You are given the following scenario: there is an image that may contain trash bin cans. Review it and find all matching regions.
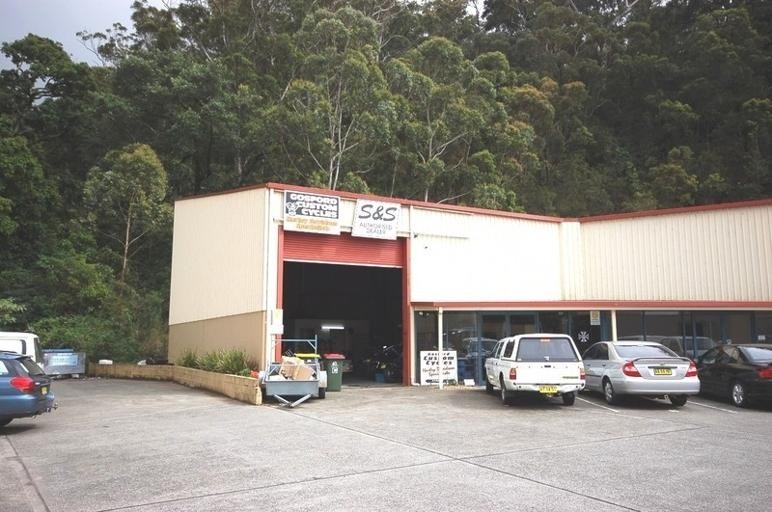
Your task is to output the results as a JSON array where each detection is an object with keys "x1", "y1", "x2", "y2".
[{"x1": 323, "y1": 354, "x2": 346, "y2": 392}]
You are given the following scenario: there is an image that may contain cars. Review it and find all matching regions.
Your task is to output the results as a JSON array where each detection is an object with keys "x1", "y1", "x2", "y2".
[
  {"x1": 0, "y1": 350, "x2": 58, "y2": 429},
  {"x1": 484, "y1": 333, "x2": 772, "y2": 408},
  {"x1": 461, "y1": 337, "x2": 497, "y2": 357}
]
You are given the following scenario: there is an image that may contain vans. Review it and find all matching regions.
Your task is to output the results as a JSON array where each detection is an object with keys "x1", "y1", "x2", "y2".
[{"x1": 0, "y1": 331, "x2": 45, "y2": 372}]
